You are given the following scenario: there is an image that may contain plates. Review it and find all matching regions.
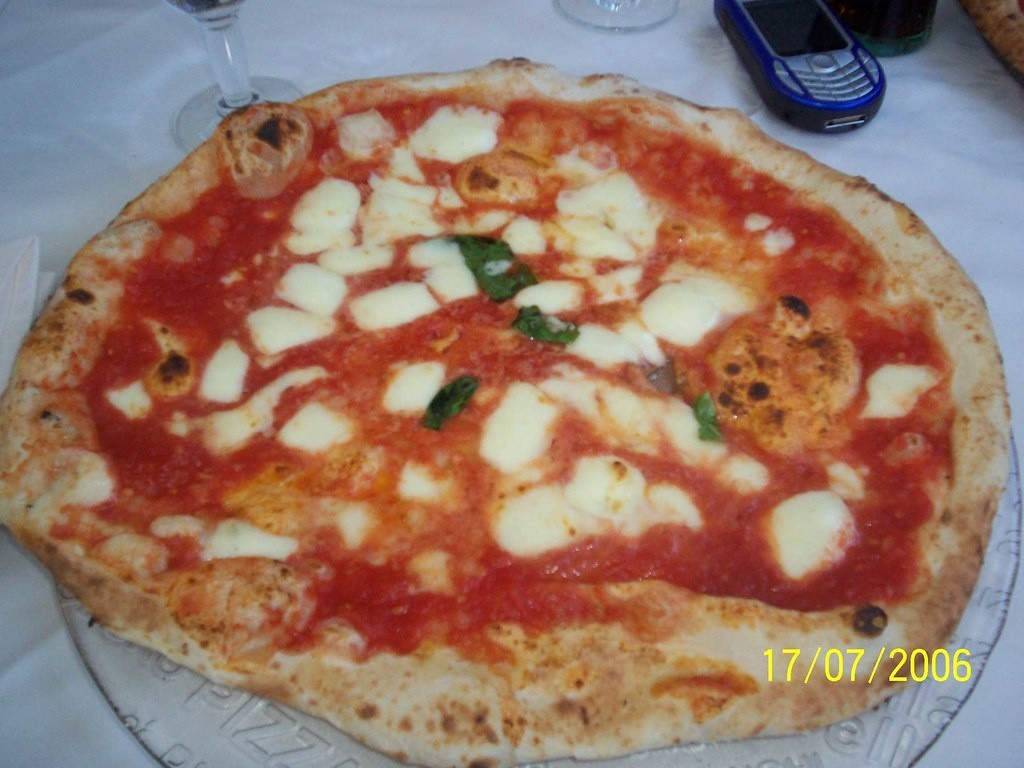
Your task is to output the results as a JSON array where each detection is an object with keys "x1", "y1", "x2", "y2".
[{"x1": 52, "y1": 425, "x2": 1022, "y2": 768}]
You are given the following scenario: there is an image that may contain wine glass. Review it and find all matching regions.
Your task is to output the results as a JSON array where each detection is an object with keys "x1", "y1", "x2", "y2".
[{"x1": 165, "y1": 0, "x2": 303, "y2": 157}]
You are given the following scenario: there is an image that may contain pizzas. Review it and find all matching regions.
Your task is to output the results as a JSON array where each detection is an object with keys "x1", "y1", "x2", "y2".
[
  {"x1": 961, "y1": 0, "x2": 1024, "y2": 76},
  {"x1": 1, "y1": 60, "x2": 1011, "y2": 765}
]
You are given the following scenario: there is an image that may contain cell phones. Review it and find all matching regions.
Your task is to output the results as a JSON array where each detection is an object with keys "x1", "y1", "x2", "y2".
[
  {"x1": 824, "y1": 0, "x2": 935, "y2": 56},
  {"x1": 714, "y1": 0, "x2": 887, "y2": 134}
]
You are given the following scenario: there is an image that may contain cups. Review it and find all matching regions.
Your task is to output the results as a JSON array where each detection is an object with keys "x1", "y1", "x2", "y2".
[{"x1": 828, "y1": 0, "x2": 939, "y2": 60}]
